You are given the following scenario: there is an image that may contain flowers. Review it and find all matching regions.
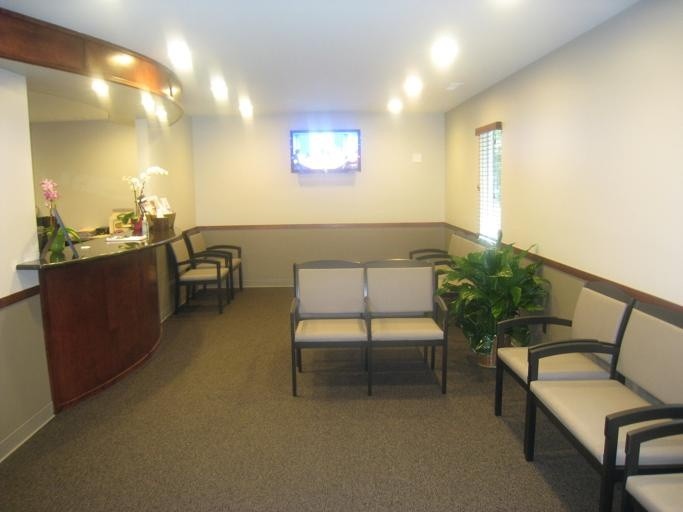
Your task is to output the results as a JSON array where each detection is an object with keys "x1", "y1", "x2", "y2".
[
  {"x1": 115, "y1": 165, "x2": 168, "y2": 225},
  {"x1": 35, "y1": 177, "x2": 82, "y2": 244}
]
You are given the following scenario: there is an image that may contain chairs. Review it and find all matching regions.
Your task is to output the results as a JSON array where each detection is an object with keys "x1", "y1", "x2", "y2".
[
  {"x1": 166, "y1": 227, "x2": 243, "y2": 316},
  {"x1": 494, "y1": 281, "x2": 634, "y2": 460},
  {"x1": 365, "y1": 259, "x2": 449, "y2": 398},
  {"x1": 408, "y1": 235, "x2": 488, "y2": 328},
  {"x1": 289, "y1": 259, "x2": 371, "y2": 396}
]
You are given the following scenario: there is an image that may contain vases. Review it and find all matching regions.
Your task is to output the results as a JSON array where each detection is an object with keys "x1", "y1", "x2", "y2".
[
  {"x1": 129, "y1": 216, "x2": 143, "y2": 236},
  {"x1": 44, "y1": 231, "x2": 64, "y2": 252}
]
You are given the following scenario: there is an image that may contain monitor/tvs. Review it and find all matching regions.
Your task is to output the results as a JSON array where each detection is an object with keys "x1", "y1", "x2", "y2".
[{"x1": 290, "y1": 128, "x2": 362, "y2": 174}]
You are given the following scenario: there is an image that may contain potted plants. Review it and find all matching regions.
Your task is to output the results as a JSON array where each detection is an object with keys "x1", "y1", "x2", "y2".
[{"x1": 433, "y1": 228, "x2": 554, "y2": 368}]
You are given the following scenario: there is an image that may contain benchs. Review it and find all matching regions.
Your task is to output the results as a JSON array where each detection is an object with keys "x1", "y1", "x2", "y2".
[{"x1": 525, "y1": 300, "x2": 683, "y2": 510}]
